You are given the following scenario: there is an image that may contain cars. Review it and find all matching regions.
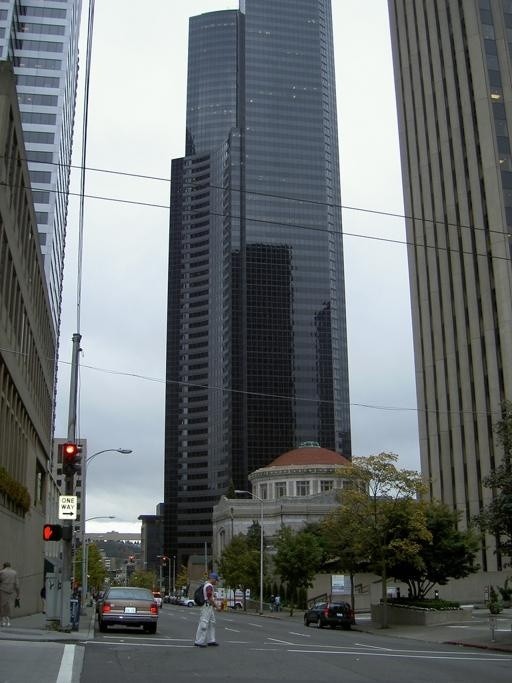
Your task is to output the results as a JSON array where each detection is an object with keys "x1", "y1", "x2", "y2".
[
  {"x1": 95, "y1": 590, "x2": 105, "y2": 600},
  {"x1": 99, "y1": 585, "x2": 158, "y2": 634},
  {"x1": 96, "y1": 597, "x2": 104, "y2": 609},
  {"x1": 153, "y1": 591, "x2": 199, "y2": 608}
]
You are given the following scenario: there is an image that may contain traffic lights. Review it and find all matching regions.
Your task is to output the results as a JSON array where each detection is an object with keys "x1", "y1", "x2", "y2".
[
  {"x1": 41, "y1": 522, "x2": 63, "y2": 541},
  {"x1": 60, "y1": 442, "x2": 79, "y2": 475}
]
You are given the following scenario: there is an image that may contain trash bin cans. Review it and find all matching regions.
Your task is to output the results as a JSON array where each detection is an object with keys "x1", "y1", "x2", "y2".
[{"x1": 70, "y1": 600, "x2": 80, "y2": 631}]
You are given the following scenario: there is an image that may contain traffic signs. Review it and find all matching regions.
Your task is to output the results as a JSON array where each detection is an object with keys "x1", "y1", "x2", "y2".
[{"x1": 57, "y1": 494, "x2": 78, "y2": 521}]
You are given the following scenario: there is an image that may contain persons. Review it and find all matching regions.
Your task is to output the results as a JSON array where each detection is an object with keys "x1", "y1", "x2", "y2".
[
  {"x1": 194, "y1": 573, "x2": 221, "y2": 648},
  {"x1": 269, "y1": 595, "x2": 275, "y2": 613},
  {"x1": 0, "y1": 561, "x2": 20, "y2": 627},
  {"x1": 274, "y1": 594, "x2": 280, "y2": 613}
]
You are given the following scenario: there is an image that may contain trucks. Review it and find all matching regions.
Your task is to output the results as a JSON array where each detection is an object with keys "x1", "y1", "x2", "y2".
[{"x1": 212, "y1": 587, "x2": 251, "y2": 609}]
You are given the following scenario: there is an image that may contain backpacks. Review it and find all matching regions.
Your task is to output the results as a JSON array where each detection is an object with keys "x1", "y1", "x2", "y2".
[{"x1": 194, "y1": 584, "x2": 206, "y2": 606}]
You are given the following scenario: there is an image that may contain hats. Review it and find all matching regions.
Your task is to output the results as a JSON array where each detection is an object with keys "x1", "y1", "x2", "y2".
[{"x1": 210, "y1": 573, "x2": 221, "y2": 581}]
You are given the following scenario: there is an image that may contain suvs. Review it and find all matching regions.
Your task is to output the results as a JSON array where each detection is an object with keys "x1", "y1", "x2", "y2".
[{"x1": 303, "y1": 599, "x2": 353, "y2": 629}]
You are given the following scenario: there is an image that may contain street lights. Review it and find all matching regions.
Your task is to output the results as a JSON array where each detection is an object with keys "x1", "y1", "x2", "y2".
[
  {"x1": 85, "y1": 534, "x2": 103, "y2": 594},
  {"x1": 80, "y1": 447, "x2": 133, "y2": 601},
  {"x1": 234, "y1": 489, "x2": 265, "y2": 615},
  {"x1": 156, "y1": 555, "x2": 171, "y2": 596},
  {"x1": 72, "y1": 515, "x2": 116, "y2": 600}
]
